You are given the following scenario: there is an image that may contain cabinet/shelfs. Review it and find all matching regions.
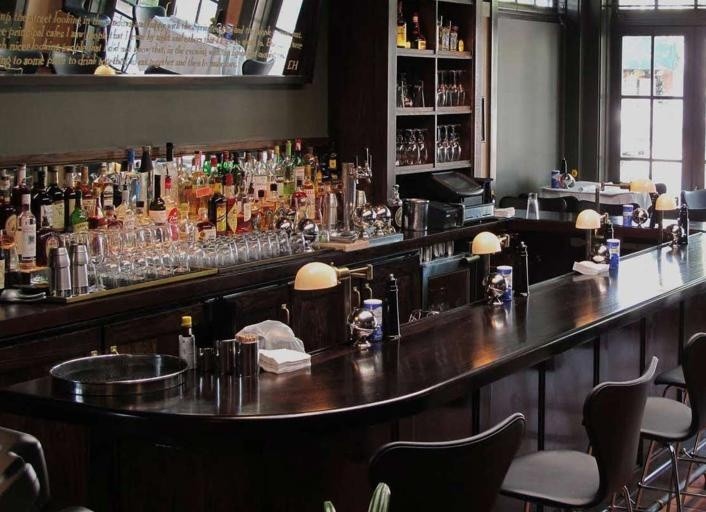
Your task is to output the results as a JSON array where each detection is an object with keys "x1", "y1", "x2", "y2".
[
  {"x1": 324, "y1": 0, "x2": 478, "y2": 230},
  {"x1": 0, "y1": 252, "x2": 422, "y2": 512}
]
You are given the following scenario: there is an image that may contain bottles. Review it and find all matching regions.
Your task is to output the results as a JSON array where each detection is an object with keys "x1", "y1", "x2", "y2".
[
  {"x1": 512, "y1": 241, "x2": 531, "y2": 297},
  {"x1": 387, "y1": 185, "x2": 403, "y2": 233},
  {"x1": 678, "y1": 203, "x2": 689, "y2": 245},
  {"x1": 396, "y1": 1, "x2": 428, "y2": 49},
  {"x1": 0, "y1": 137, "x2": 340, "y2": 294},
  {"x1": 438, "y1": 15, "x2": 465, "y2": 52},
  {"x1": 395, "y1": 71, "x2": 426, "y2": 107},
  {"x1": 177, "y1": 315, "x2": 196, "y2": 370},
  {"x1": 382, "y1": 273, "x2": 401, "y2": 340},
  {"x1": 526, "y1": 192, "x2": 542, "y2": 220},
  {"x1": 560, "y1": 157, "x2": 567, "y2": 174}
]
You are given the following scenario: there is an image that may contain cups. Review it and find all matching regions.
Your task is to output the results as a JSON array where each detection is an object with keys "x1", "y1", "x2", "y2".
[
  {"x1": 364, "y1": 298, "x2": 383, "y2": 342},
  {"x1": 496, "y1": 265, "x2": 514, "y2": 302},
  {"x1": 198, "y1": 339, "x2": 259, "y2": 379},
  {"x1": 48, "y1": 221, "x2": 306, "y2": 299},
  {"x1": 622, "y1": 205, "x2": 634, "y2": 228},
  {"x1": 606, "y1": 238, "x2": 621, "y2": 270},
  {"x1": 223, "y1": 24, "x2": 233, "y2": 47},
  {"x1": 550, "y1": 169, "x2": 560, "y2": 188}
]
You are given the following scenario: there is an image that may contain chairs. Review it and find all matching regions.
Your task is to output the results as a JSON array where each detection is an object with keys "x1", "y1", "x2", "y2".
[{"x1": 0, "y1": 0, "x2": 276, "y2": 75}]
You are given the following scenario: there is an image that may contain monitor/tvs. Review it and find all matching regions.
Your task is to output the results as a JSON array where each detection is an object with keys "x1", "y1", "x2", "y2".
[{"x1": 431, "y1": 169, "x2": 485, "y2": 195}]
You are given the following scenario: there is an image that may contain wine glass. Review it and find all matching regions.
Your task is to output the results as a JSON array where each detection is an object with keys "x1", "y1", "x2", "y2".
[
  {"x1": 437, "y1": 69, "x2": 467, "y2": 107},
  {"x1": 436, "y1": 123, "x2": 465, "y2": 162},
  {"x1": 395, "y1": 127, "x2": 432, "y2": 166},
  {"x1": 351, "y1": 204, "x2": 391, "y2": 238}
]
